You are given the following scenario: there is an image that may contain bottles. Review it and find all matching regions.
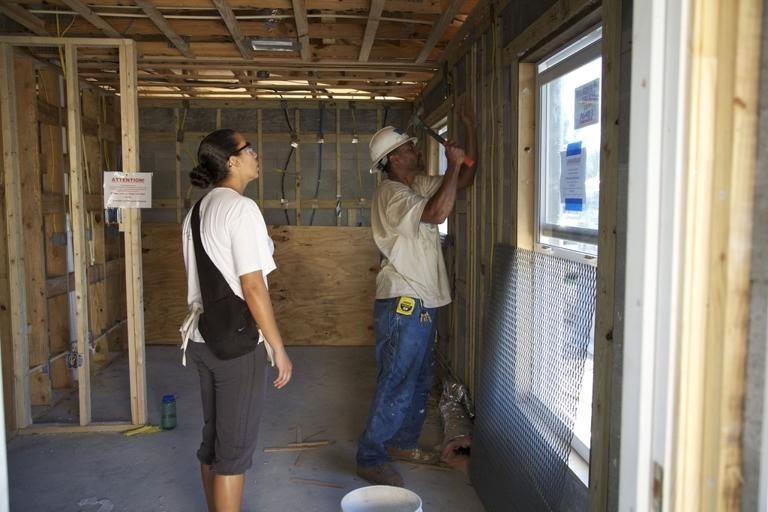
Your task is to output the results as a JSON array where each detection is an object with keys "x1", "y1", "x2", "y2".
[{"x1": 160, "y1": 394, "x2": 178, "y2": 431}]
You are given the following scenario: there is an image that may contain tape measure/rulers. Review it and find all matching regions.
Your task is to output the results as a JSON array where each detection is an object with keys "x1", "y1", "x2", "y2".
[{"x1": 395, "y1": 296, "x2": 416, "y2": 316}]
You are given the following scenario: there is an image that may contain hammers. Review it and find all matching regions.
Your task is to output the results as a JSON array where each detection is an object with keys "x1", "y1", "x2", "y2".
[{"x1": 406, "y1": 106, "x2": 475, "y2": 169}]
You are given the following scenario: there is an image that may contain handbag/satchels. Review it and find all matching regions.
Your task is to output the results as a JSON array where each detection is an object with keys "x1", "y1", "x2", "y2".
[{"x1": 188, "y1": 193, "x2": 260, "y2": 361}]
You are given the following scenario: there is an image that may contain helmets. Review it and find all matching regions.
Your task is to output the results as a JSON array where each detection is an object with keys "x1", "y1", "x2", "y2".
[{"x1": 366, "y1": 125, "x2": 419, "y2": 173}]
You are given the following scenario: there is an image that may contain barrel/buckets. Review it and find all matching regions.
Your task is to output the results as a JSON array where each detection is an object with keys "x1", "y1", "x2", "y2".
[{"x1": 338, "y1": 484, "x2": 424, "y2": 512}]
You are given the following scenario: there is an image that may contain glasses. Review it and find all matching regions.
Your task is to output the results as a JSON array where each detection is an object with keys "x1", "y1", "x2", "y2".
[{"x1": 227, "y1": 141, "x2": 251, "y2": 160}]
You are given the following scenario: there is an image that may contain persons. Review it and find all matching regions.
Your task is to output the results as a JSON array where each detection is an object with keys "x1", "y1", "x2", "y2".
[
  {"x1": 355, "y1": 95, "x2": 481, "y2": 488},
  {"x1": 179, "y1": 129, "x2": 294, "y2": 512}
]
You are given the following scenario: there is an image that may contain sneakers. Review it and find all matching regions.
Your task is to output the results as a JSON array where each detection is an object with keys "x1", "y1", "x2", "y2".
[
  {"x1": 357, "y1": 461, "x2": 403, "y2": 490},
  {"x1": 386, "y1": 443, "x2": 441, "y2": 465}
]
